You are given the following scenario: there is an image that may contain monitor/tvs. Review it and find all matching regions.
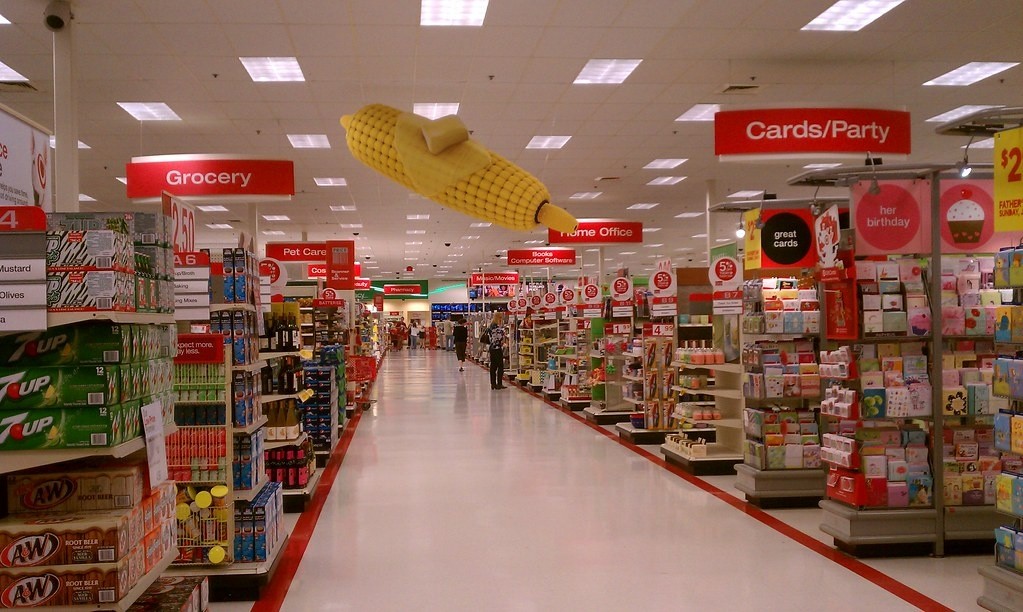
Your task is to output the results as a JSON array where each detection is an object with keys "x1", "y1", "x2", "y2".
[{"x1": 432, "y1": 303, "x2": 508, "y2": 326}]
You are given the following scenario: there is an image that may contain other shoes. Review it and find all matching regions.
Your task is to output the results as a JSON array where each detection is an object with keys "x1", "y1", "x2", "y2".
[
  {"x1": 459, "y1": 369, "x2": 464, "y2": 371},
  {"x1": 461, "y1": 367, "x2": 462, "y2": 372},
  {"x1": 491, "y1": 384, "x2": 501, "y2": 390},
  {"x1": 497, "y1": 384, "x2": 507, "y2": 389}
]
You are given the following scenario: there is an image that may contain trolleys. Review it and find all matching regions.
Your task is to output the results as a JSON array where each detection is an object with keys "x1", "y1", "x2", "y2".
[{"x1": 389, "y1": 327, "x2": 408, "y2": 352}]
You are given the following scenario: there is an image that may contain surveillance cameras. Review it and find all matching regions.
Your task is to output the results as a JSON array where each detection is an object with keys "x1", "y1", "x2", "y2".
[
  {"x1": 433, "y1": 265, "x2": 437, "y2": 267},
  {"x1": 463, "y1": 272, "x2": 466, "y2": 273},
  {"x1": 43, "y1": 0, "x2": 71, "y2": 31},
  {"x1": 445, "y1": 243, "x2": 451, "y2": 246},
  {"x1": 353, "y1": 233, "x2": 359, "y2": 235},
  {"x1": 495, "y1": 255, "x2": 500, "y2": 257},
  {"x1": 366, "y1": 257, "x2": 370, "y2": 259},
  {"x1": 396, "y1": 272, "x2": 400, "y2": 279}
]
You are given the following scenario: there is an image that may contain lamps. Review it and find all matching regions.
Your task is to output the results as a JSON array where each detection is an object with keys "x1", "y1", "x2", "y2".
[
  {"x1": 868, "y1": 150, "x2": 881, "y2": 194},
  {"x1": 735, "y1": 210, "x2": 746, "y2": 238},
  {"x1": 755, "y1": 189, "x2": 767, "y2": 229},
  {"x1": 956, "y1": 135, "x2": 976, "y2": 178},
  {"x1": 810, "y1": 182, "x2": 822, "y2": 216}
]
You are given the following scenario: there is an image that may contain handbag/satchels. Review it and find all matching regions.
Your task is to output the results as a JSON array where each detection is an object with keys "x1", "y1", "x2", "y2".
[{"x1": 480, "y1": 328, "x2": 490, "y2": 344}]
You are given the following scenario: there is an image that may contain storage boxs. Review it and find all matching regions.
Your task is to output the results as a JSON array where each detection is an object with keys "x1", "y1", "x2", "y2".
[
  {"x1": 519, "y1": 250, "x2": 1023, "y2": 576},
  {"x1": 2, "y1": 208, "x2": 371, "y2": 612}
]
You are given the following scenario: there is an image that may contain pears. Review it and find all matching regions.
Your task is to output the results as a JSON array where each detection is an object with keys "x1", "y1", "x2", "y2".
[{"x1": 864, "y1": 395, "x2": 883, "y2": 416}]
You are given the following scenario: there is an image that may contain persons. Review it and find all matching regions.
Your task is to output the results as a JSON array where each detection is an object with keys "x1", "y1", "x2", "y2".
[
  {"x1": 389, "y1": 317, "x2": 426, "y2": 349},
  {"x1": 488, "y1": 312, "x2": 508, "y2": 390},
  {"x1": 486, "y1": 285, "x2": 508, "y2": 296},
  {"x1": 444, "y1": 316, "x2": 455, "y2": 352},
  {"x1": 453, "y1": 319, "x2": 467, "y2": 372}
]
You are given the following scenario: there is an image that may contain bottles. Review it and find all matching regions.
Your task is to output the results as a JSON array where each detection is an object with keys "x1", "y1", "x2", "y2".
[
  {"x1": 261, "y1": 355, "x2": 303, "y2": 395},
  {"x1": 261, "y1": 399, "x2": 303, "y2": 442},
  {"x1": 258, "y1": 312, "x2": 301, "y2": 353},
  {"x1": 264, "y1": 438, "x2": 316, "y2": 489}
]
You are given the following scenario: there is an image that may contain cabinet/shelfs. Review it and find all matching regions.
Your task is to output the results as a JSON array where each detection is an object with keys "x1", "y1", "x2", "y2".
[
  {"x1": 660, "y1": 311, "x2": 748, "y2": 476},
  {"x1": 615, "y1": 337, "x2": 669, "y2": 446},
  {"x1": 786, "y1": 161, "x2": 1018, "y2": 557},
  {"x1": 259, "y1": 350, "x2": 321, "y2": 515},
  {"x1": 435, "y1": 311, "x2": 594, "y2": 412},
  {"x1": 977, "y1": 247, "x2": 1023, "y2": 612},
  {"x1": 0, "y1": 310, "x2": 181, "y2": 612},
  {"x1": 733, "y1": 281, "x2": 826, "y2": 509},
  {"x1": 583, "y1": 334, "x2": 641, "y2": 424},
  {"x1": 168, "y1": 261, "x2": 288, "y2": 600}
]
